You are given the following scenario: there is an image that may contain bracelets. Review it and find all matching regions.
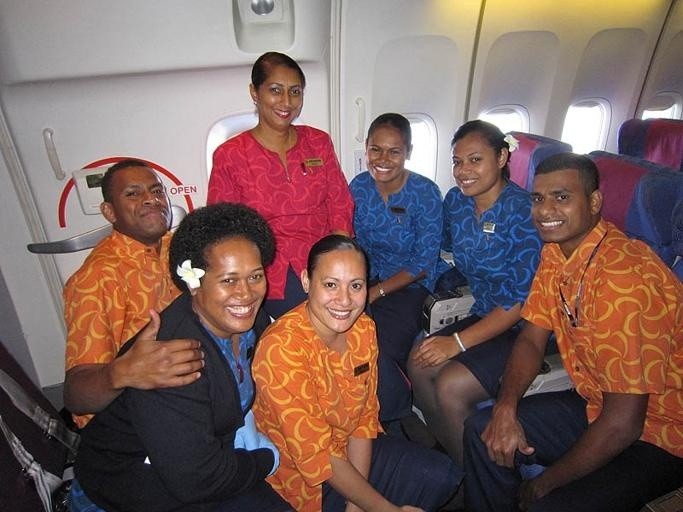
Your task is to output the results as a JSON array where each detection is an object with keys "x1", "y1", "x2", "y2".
[
  {"x1": 375, "y1": 282, "x2": 387, "y2": 297},
  {"x1": 451, "y1": 332, "x2": 467, "y2": 354}
]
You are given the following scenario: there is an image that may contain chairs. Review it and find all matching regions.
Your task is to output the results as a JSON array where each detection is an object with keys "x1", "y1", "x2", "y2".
[
  {"x1": 414, "y1": 132, "x2": 572, "y2": 409},
  {"x1": 478, "y1": 148, "x2": 682, "y2": 486},
  {"x1": 617, "y1": 117, "x2": 682, "y2": 172}
]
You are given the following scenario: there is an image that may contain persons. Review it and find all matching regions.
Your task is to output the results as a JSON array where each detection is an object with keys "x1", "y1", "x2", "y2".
[
  {"x1": 346, "y1": 109, "x2": 447, "y2": 445},
  {"x1": 202, "y1": 48, "x2": 355, "y2": 331},
  {"x1": 66, "y1": 200, "x2": 294, "y2": 511},
  {"x1": 243, "y1": 229, "x2": 467, "y2": 512},
  {"x1": 58, "y1": 158, "x2": 209, "y2": 430},
  {"x1": 460, "y1": 152, "x2": 682, "y2": 512},
  {"x1": 403, "y1": 119, "x2": 548, "y2": 472}
]
